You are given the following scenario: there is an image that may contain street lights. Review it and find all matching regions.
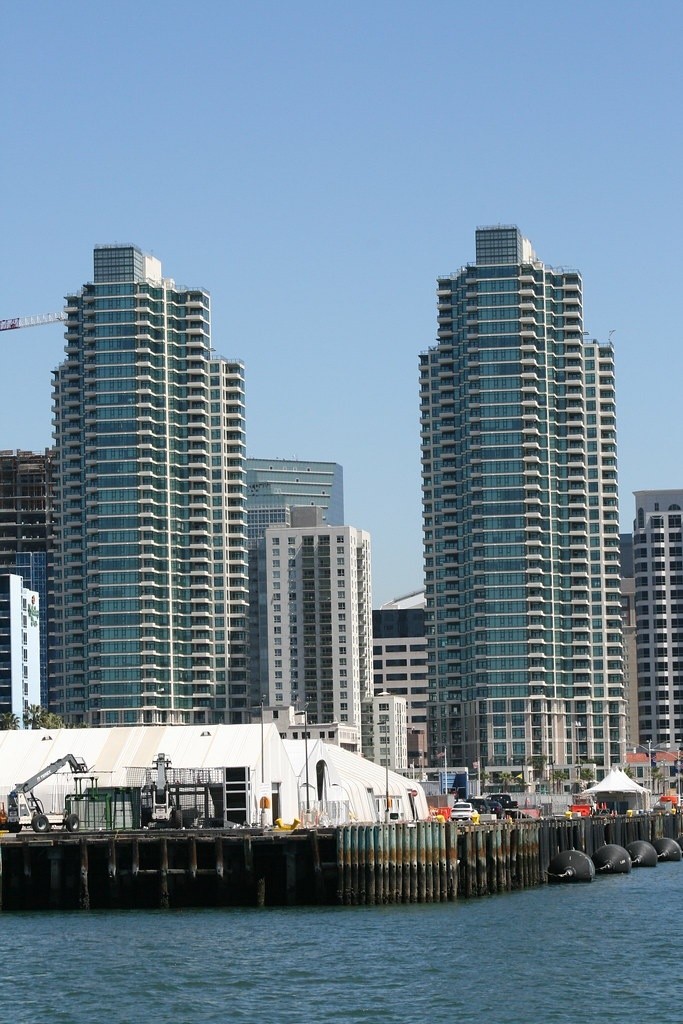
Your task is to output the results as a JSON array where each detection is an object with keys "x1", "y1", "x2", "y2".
[
  {"x1": 294, "y1": 709, "x2": 312, "y2": 825},
  {"x1": 574, "y1": 720, "x2": 582, "y2": 781},
  {"x1": 376, "y1": 720, "x2": 390, "y2": 823},
  {"x1": 443, "y1": 745, "x2": 449, "y2": 795},
  {"x1": 617, "y1": 738, "x2": 682, "y2": 806},
  {"x1": 650, "y1": 748, "x2": 682, "y2": 810},
  {"x1": 260, "y1": 694, "x2": 267, "y2": 826}
]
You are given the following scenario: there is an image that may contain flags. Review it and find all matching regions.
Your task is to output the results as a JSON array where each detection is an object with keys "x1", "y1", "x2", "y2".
[
  {"x1": 472, "y1": 762, "x2": 478, "y2": 769},
  {"x1": 437, "y1": 750, "x2": 445, "y2": 757}
]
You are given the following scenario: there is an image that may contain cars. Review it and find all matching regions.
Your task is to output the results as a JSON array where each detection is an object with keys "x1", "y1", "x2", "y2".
[{"x1": 449, "y1": 793, "x2": 532, "y2": 821}]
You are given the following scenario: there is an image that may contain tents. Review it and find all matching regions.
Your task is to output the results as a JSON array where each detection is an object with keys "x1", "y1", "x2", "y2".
[{"x1": 579, "y1": 766, "x2": 651, "y2": 814}]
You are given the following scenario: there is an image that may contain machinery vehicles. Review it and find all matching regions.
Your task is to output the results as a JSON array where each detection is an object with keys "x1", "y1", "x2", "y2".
[
  {"x1": 6, "y1": 753, "x2": 89, "y2": 833},
  {"x1": 141, "y1": 754, "x2": 184, "y2": 830}
]
